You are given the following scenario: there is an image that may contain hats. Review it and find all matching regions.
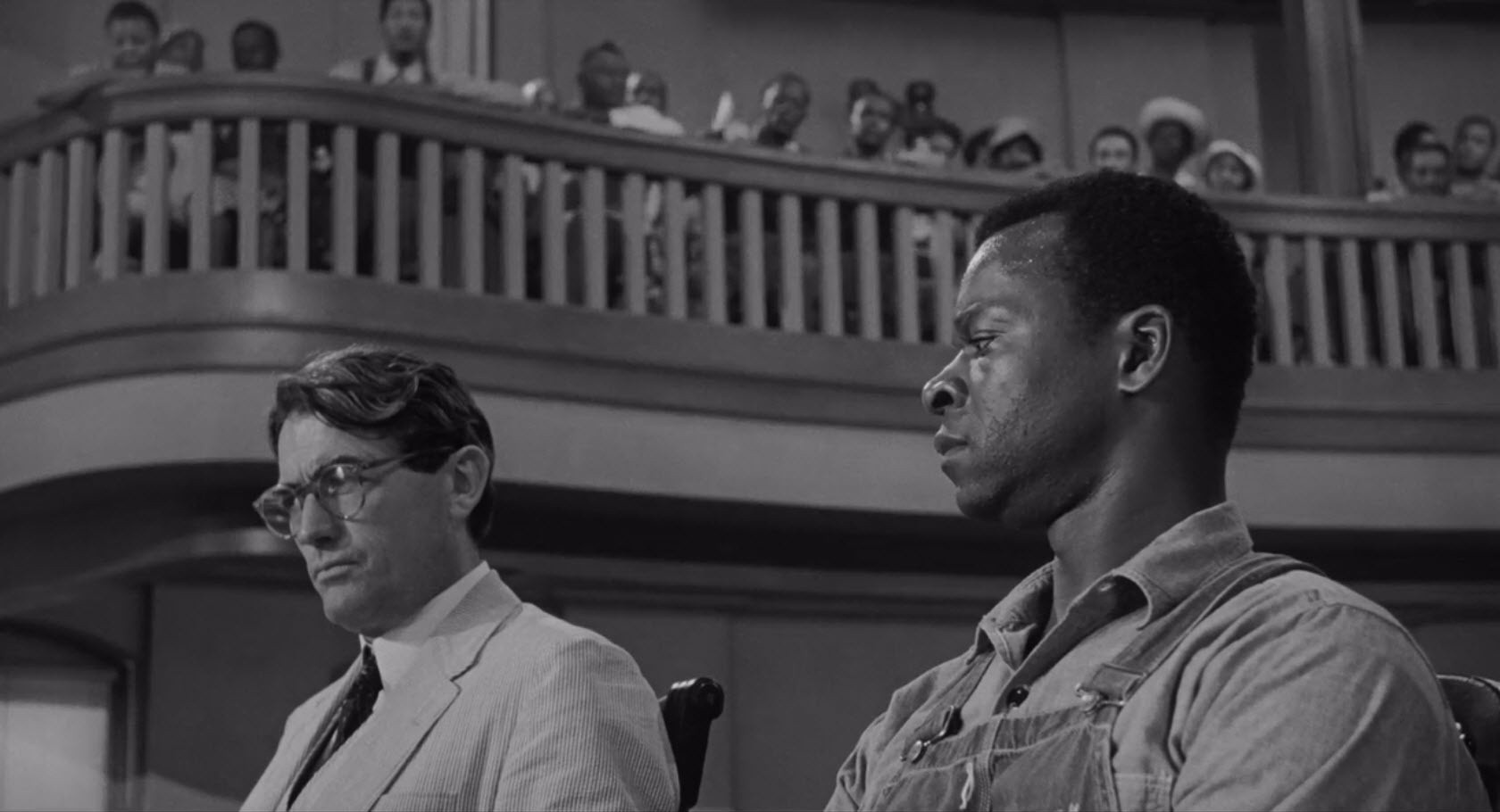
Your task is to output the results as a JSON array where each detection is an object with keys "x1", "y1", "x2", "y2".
[
  {"x1": 1137, "y1": 97, "x2": 1206, "y2": 148},
  {"x1": 981, "y1": 117, "x2": 1046, "y2": 162}
]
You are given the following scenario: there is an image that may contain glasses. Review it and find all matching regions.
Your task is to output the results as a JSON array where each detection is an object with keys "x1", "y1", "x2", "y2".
[{"x1": 252, "y1": 445, "x2": 434, "y2": 541}]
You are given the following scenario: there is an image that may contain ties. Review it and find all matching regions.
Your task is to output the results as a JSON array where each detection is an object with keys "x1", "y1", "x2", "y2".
[{"x1": 330, "y1": 659, "x2": 383, "y2": 756}]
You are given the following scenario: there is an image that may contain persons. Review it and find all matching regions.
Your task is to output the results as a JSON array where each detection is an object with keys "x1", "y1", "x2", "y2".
[
  {"x1": 816, "y1": 170, "x2": 1495, "y2": 812},
  {"x1": 35, "y1": 1, "x2": 1500, "y2": 383},
  {"x1": 222, "y1": 340, "x2": 686, "y2": 811}
]
